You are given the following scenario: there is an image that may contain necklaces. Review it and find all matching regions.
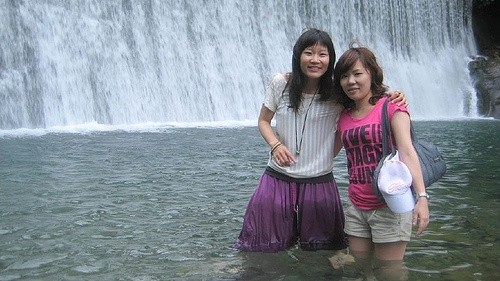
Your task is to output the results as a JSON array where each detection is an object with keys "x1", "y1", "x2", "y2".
[{"x1": 295, "y1": 85, "x2": 319, "y2": 155}]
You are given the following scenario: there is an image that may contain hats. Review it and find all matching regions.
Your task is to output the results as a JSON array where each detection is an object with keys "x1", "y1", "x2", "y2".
[{"x1": 378, "y1": 150, "x2": 414, "y2": 213}]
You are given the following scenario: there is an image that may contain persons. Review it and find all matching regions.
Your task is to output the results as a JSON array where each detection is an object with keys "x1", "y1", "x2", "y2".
[
  {"x1": 239, "y1": 28, "x2": 409, "y2": 242},
  {"x1": 334, "y1": 47, "x2": 429, "y2": 260}
]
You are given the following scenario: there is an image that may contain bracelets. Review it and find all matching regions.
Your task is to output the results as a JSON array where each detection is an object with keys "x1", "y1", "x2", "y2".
[
  {"x1": 416, "y1": 192, "x2": 429, "y2": 200},
  {"x1": 271, "y1": 141, "x2": 281, "y2": 153}
]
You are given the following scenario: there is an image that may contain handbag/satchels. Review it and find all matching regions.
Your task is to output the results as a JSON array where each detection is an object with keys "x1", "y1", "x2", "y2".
[{"x1": 371, "y1": 99, "x2": 446, "y2": 204}]
